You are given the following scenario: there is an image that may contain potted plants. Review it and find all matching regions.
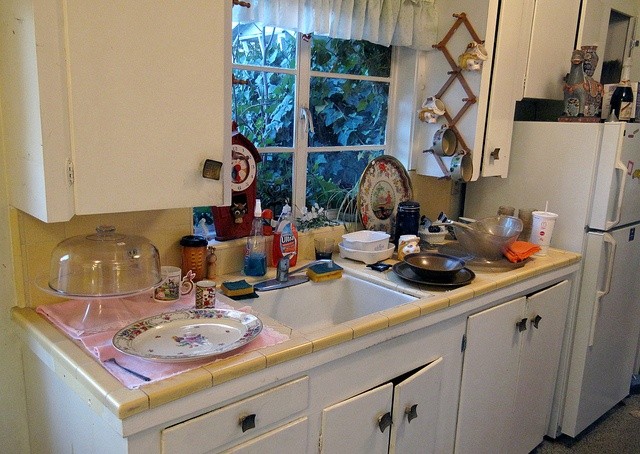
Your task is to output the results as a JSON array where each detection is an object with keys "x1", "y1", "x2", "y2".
[{"x1": 326, "y1": 187, "x2": 362, "y2": 223}]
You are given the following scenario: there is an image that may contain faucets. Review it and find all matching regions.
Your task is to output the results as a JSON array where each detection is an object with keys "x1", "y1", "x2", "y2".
[{"x1": 272, "y1": 251, "x2": 333, "y2": 282}]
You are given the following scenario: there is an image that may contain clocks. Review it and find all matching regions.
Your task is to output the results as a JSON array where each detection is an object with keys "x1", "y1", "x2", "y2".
[{"x1": 211, "y1": 121, "x2": 263, "y2": 242}]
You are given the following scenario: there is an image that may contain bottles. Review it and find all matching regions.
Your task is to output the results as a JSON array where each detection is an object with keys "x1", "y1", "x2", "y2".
[
  {"x1": 394, "y1": 200, "x2": 421, "y2": 254},
  {"x1": 608, "y1": 56, "x2": 634, "y2": 122}
]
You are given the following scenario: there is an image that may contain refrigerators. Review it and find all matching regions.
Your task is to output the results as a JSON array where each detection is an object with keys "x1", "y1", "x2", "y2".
[{"x1": 460, "y1": 121, "x2": 639, "y2": 445}]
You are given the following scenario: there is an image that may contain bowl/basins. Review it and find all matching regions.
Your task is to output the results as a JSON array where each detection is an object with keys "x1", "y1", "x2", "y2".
[
  {"x1": 453, "y1": 215, "x2": 524, "y2": 260},
  {"x1": 402, "y1": 251, "x2": 466, "y2": 280},
  {"x1": 340, "y1": 230, "x2": 392, "y2": 251},
  {"x1": 338, "y1": 242, "x2": 396, "y2": 265}
]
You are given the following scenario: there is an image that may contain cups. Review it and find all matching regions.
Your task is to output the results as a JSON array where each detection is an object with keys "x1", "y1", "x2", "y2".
[
  {"x1": 151, "y1": 264, "x2": 193, "y2": 304},
  {"x1": 314, "y1": 236, "x2": 335, "y2": 261},
  {"x1": 195, "y1": 280, "x2": 217, "y2": 310},
  {"x1": 432, "y1": 124, "x2": 458, "y2": 157},
  {"x1": 529, "y1": 210, "x2": 559, "y2": 256},
  {"x1": 417, "y1": 96, "x2": 446, "y2": 125},
  {"x1": 449, "y1": 149, "x2": 474, "y2": 184},
  {"x1": 457, "y1": 41, "x2": 489, "y2": 73}
]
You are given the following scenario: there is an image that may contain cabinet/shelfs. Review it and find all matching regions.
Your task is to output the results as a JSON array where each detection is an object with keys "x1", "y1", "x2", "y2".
[
  {"x1": 415, "y1": 0, "x2": 538, "y2": 180},
  {"x1": 523, "y1": 0, "x2": 617, "y2": 102},
  {"x1": 0, "y1": 0, "x2": 234, "y2": 226},
  {"x1": 452, "y1": 277, "x2": 574, "y2": 454},
  {"x1": 318, "y1": 355, "x2": 445, "y2": 453},
  {"x1": 156, "y1": 373, "x2": 312, "y2": 453}
]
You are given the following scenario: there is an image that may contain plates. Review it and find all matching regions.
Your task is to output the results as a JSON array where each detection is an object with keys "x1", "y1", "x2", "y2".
[
  {"x1": 437, "y1": 243, "x2": 532, "y2": 267},
  {"x1": 111, "y1": 307, "x2": 265, "y2": 364},
  {"x1": 392, "y1": 259, "x2": 476, "y2": 286},
  {"x1": 356, "y1": 155, "x2": 415, "y2": 242}
]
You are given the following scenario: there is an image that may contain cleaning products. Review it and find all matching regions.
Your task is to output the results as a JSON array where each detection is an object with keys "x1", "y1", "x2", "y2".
[
  {"x1": 242, "y1": 199, "x2": 270, "y2": 274},
  {"x1": 272, "y1": 205, "x2": 299, "y2": 268}
]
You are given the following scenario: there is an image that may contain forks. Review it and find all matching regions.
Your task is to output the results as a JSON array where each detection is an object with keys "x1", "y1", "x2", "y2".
[{"x1": 104, "y1": 356, "x2": 151, "y2": 382}]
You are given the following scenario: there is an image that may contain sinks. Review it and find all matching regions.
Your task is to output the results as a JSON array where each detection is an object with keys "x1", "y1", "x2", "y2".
[{"x1": 233, "y1": 275, "x2": 420, "y2": 329}]
